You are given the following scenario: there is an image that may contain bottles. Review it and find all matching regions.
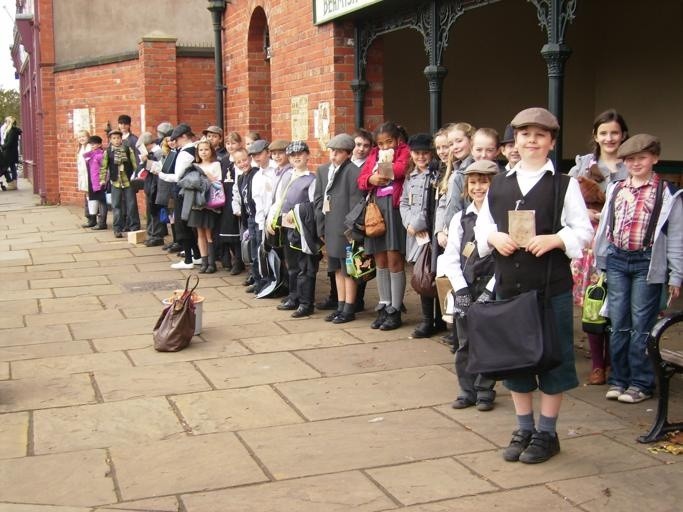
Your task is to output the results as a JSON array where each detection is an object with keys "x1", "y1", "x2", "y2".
[{"x1": 344, "y1": 245, "x2": 355, "y2": 275}]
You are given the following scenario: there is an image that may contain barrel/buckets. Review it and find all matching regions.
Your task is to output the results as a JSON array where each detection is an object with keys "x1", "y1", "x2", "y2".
[
  {"x1": 162, "y1": 296, "x2": 205, "y2": 336},
  {"x1": 106, "y1": 193, "x2": 110, "y2": 204},
  {"x1": 88, "y1": 200, "x2": 98, "y2": 215}
]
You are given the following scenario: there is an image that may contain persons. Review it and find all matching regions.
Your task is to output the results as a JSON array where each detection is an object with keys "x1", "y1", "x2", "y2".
[
  {"x1": 0, "y1": 115, "x2": 22, "y2": 192},
  {"x1": 593, "y1": 130, "x2": 683, "y2": 403},
  {"x1": 566, "y1": 110, "x2": 630, "y2": 384},
  {"x1": 471, "y1": 106, "x2": 596, "y2": 467},
  {"x1": 221, "y1": 131, "x2": 376, "y2": 324},
  {"x1": 78, "y1": 114, "x2": 227, "y2": 273},
  {"x1": 440, "y1": 159, "x2": 501, "y2": 411},
  {"x1": 357, "y1": 122, "x2": 475, "y2": 345}
]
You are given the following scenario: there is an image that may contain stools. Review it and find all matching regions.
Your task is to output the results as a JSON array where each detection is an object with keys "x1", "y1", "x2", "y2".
[{"x1": 636, "y1": 309, "x2": 682, "y2": 446}]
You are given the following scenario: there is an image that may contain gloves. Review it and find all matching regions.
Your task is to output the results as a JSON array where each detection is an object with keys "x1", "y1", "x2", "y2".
[
  {"x1": 454, "y1": 286, "x2": 474, "y2": 320},
  {"x1": 475, "y1": 288, "x2": 493, "y2": 303}
]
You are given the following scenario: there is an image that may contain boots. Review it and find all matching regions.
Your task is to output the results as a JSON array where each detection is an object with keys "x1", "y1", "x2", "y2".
[
  {"x1": 379, "y1": 302, "x2": 407, "y2": 330},
  {"x1": 371, "y1": 302, "x2": 392, "y2": 329}
]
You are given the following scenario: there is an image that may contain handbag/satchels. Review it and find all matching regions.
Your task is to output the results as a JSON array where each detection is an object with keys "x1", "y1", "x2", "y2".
[
  {"x1": 202, "y1": 181, "x2": 225, "y2": 208},
  {"x1": 442, "y1": 288, "x2": 456, "y2": 324},
  {"x1": 240, "y1": 235, "x2": 253, "y2": 263},
  {"x1": 581, "y1": 270, "x2": 608, "y2": 335},
  {"x1": 364, "y1": 195, "x2": 386, "y2": 239},
  {"x1": 343, "y1": 190, "x2": 373, "y2": 236},
  {"x1": 151, "y1": 274, "x2": 199, "y2": 352},
  {"x1": 345, "y1": 239, "x2": 376, "y2": 286},
  {"x1": 263, "y1": 225, "x2": 286, "y2": 249},
  {"x1": 410, "y1": 242, "x2": 438, "y2": 299},
  {"x1": 159, "y1": 205, "x2": 175, "y2": 224},
  {"x1": 465, "y1": 289, "x2": 544, "y2": 374}
]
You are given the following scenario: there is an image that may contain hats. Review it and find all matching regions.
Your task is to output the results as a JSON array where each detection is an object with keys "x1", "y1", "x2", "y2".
[
  {"x1": 462, "y1": 107, "x2": 561, "y2": 175},
  {"x1": 616, "y1": 134, "x2": 660, "y2": 159},
  {"x1": 408, "y1": 133, "x2": 436, "y2": 151},
  {"x1": 135, "y1": 122, "x2": 223, "y2": 148},
  {"x1": 118, "y1": 115, "x2": 131, "y2": 124},
  {"x1": 88, "y1": 136, "x2": 102, "y2": 144},
  {"x1": 107, "y1": 129, "x2": 122, "y2": 138},
  {"x1": 326, "y1": 134, "x2": 355, "y2": 151},
  {"x1": 247, "y1": 140, "x2": 309, "y2": 157}
]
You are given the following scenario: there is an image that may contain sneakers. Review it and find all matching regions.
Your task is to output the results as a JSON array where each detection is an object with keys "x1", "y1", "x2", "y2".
[
  {"x1": 242, "y1": 273, "x2": 314, "y2": 318},
  {"x1": 519, "y1": 430, "x2": 560, "y2": 464},
  {"x1": 589, "y1": 365, "x2": 652, "y2": 403},
  {"x1": 81, "y1": 222, "x2": 140, "y2": 238},
  {"x1": 411, "y1": 318, "x2": 455, "y2": 345},
  {"x1": 313, "y1": 296, "x2": 364, "y2": 323},
  {"x1": 143, "y1": 237, "x2": 245, "y2": 275},
  {"x1": 475, "y1": 397, "x2": 493, "y2": 411},
  {"x1": 452, "y1": 396, "x2": 475, "y2": 409},
  {"x1": 504, "y1": 428, "x2": 538, "y2": 461}
]
